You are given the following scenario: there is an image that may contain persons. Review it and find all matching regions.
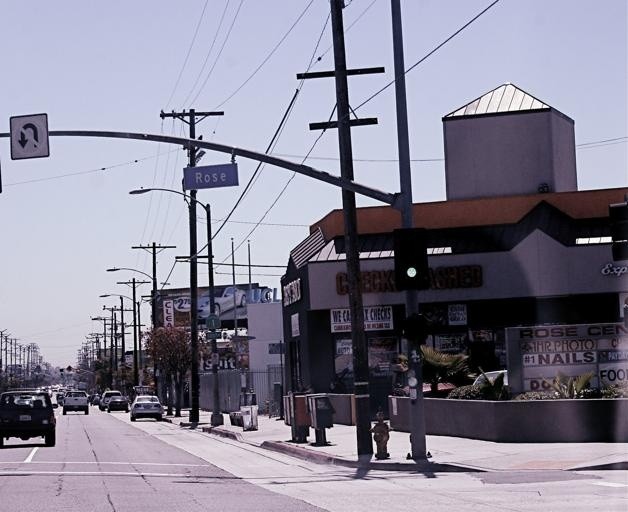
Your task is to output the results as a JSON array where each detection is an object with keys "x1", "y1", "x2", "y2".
[{"x1": 6, "y1": 396, "x2": 20, "y2": 406}]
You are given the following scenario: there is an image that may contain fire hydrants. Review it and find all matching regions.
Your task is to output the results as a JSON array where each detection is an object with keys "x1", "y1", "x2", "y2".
[{"x1": 369, "y1": 410, "x2": 392, "y2": 459}]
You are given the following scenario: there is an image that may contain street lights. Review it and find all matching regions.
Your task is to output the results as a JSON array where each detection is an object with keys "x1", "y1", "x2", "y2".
[{"x1": 76, "y1": 183, "x2": 225, "y2": 427}]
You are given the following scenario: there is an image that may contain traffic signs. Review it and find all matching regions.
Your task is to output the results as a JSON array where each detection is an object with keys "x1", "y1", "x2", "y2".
[{"x1": 184, "y1": 164, "x2": 239, "y2": 188}]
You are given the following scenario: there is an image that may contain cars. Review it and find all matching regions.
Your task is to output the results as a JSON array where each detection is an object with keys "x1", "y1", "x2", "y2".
[
  {"x1": 471, "y1": 371, "x2": 508, "y2": 389},
  {"x1": 401, "y1": 378, "x2": 453, "y2": 397},
  {"x1": 0, "y1": 384, "x2": 90, "y2": 447},
  {"x1": 194, "y1": 287, "x2": 248, "y2": 321}
]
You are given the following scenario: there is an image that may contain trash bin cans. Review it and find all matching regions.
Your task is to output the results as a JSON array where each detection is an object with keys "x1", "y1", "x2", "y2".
[{"x1": 240, "y1": 404, "x2": 259, "y2": 431}]
